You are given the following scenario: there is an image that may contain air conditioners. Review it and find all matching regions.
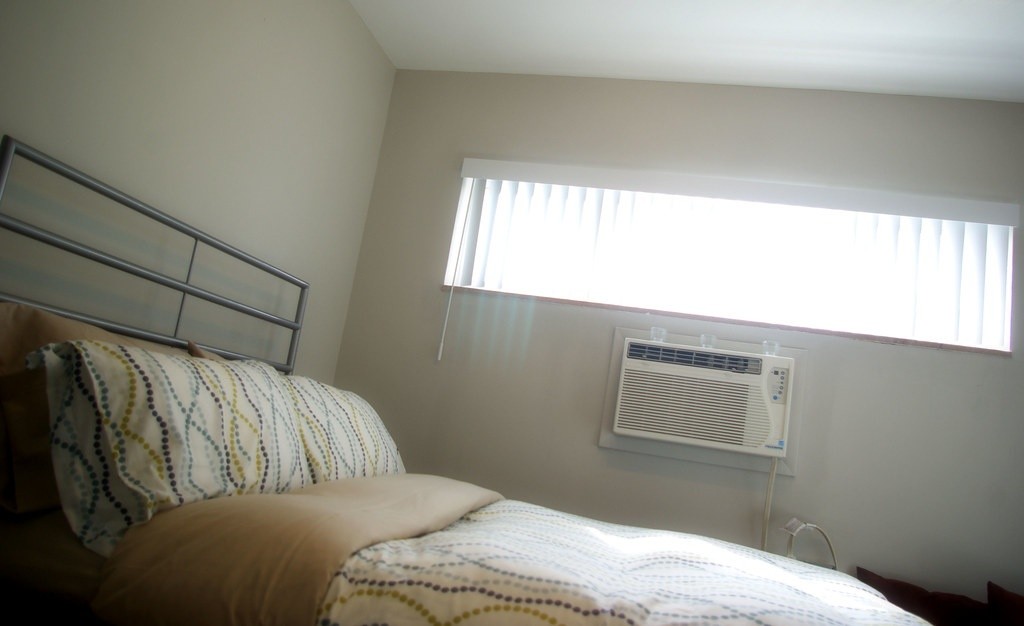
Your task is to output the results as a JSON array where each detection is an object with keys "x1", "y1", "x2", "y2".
[{"x1": 615, "y1": 336, "x2": 794, "y2": 458}]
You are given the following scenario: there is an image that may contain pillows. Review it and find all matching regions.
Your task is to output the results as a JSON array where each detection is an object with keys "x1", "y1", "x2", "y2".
[
  {"x1": 279, "y1": 373, "x2": 407, "y2": 485},
  {"x1": 187, "y1": 341, "x2": 230, "y2": 361},
  {"x1": 16, "y1": 334, "x2": 314, "y2": 561},
  {"x1": 986, "y1": 580, "x2": 1024, "y2": 626},
  {"x1": 0, "y1": 299, "x2": 194, "y2": 521},
  {"x1": 856, "y1": 567, "x2": 988, "y2": 626}
]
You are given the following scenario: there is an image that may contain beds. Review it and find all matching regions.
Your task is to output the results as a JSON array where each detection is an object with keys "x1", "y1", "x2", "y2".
[{"x1": 0, "y1": 130, "x2": 940, "y2": 626}]
